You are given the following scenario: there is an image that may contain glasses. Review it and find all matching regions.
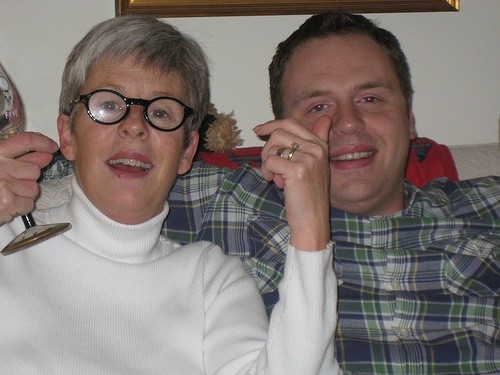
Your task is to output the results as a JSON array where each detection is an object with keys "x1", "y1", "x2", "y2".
[{"x1": 74, "y1": 89, "x2": 194, "y2": 133}]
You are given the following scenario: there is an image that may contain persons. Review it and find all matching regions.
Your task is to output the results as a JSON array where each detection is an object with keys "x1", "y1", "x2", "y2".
[
  {"x1": 0, "y1": 11, "x2": 500, "y2": 373},
  {"x1": 0, "y1": 13, "x2": 343, "y2": 374}
]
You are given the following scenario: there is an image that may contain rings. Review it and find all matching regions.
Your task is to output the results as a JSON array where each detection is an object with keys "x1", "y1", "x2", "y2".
[
  {"x1": 276, "y1": 148, "x2": 289, "y2": 157},
  {"x1": 288, "y1": 143, "x2": 298, "y2": 160}
]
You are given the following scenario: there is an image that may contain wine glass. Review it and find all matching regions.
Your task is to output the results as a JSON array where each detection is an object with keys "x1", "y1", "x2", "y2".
[{"x1": 0, "y1": 62, "x2": 72, "y2": 255}]
